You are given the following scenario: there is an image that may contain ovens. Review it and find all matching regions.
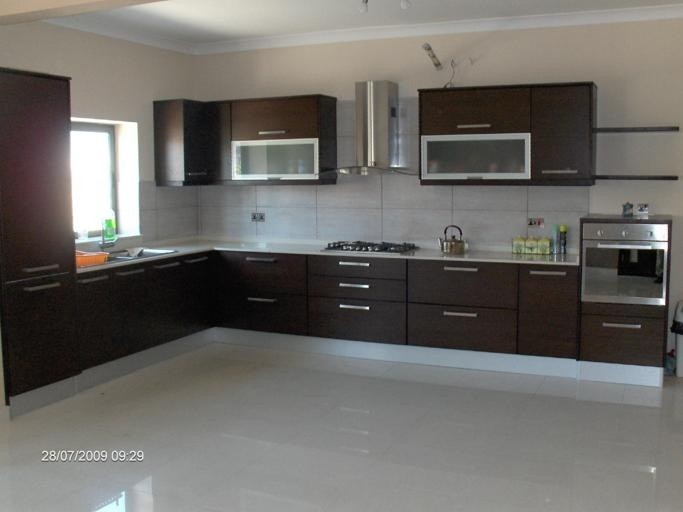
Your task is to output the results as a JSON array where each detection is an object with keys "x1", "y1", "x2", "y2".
[{"x1": 579, "y1": 221, "x2": 668, "y2": 307}]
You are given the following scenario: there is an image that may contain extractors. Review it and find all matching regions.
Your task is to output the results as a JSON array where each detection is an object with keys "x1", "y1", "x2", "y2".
[{"x1": 319, "y1": 80, "x2": 418, "y2": 178}]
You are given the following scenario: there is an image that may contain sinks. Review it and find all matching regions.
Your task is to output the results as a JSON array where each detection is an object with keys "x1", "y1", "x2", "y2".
[{"x1": 108, "y1": 246, "x2": 179, "y2": 260}]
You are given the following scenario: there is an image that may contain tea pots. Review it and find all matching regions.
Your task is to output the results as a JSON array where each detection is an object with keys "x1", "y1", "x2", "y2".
[{"x1": 437, "y1": 224, "x2": 464, "y2": 254}]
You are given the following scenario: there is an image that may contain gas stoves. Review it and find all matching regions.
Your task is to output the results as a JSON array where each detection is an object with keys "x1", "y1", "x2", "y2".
[{"x1": 324, "y1": 239, "x2": 419, "y2": 254}]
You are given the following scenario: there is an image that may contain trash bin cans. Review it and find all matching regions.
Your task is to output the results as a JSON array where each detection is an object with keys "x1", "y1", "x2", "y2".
[{"x1": 669, "y1": 301, "x2": 683, "y2": 376}]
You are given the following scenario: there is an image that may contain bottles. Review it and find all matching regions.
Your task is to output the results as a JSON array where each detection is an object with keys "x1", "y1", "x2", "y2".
[
  {"x1": 104, "y1": 206, "x2": 115, "y2": 241},
  {"x1": 551, "y1": 224, "x2": 566, "y2": 254}
]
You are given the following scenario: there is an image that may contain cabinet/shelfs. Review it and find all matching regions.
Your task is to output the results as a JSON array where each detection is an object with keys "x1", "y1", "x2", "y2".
[
  {"x1": 77, "y1": 262, "x2": 148, "y2": 372},
  {"x1": 308, "y1": 254, "x2": 406, "y2": 346},
  {"x1": 518, "y1": 262, "x2": 579, "y2": 361},
  {"x1": 0, "y1": 66, "x2": 81, "y2": 398},
  {"x1": 416, "y1": 79, "x2": 600, "y2": 187},
  {"x1": 150, "y1": 92, "x2": 337, "y2": 188},
  {"x1": 406, "y1": 259, "x2": 518, "y2": 354},
  {"x1": 148, "y1": 249, "x2": 214, "y2": 348},
  {"x1": 579, "y1": 313, "x2": 666, "y2": 368},
  {"x1": 214, "y1": 251, "x2": 308, "y2": 336}
]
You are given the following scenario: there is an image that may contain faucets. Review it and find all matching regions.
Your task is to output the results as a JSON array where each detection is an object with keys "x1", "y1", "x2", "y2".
[{"x1": 97, "y1": 228, "x2": 116, "y2": 249}]
